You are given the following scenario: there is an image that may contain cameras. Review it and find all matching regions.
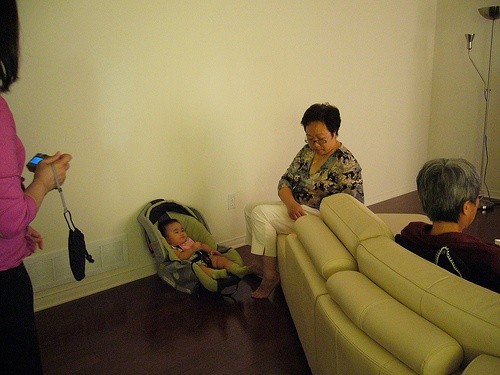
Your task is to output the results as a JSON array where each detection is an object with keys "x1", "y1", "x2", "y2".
[{"x1": 26, "y1": 153, "x2": 48, "y2": 173}]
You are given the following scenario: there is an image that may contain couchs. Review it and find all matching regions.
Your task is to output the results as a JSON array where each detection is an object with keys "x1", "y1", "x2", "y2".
[{"x1": 276, "y1": 192, "x2": 500, "y2": 375}]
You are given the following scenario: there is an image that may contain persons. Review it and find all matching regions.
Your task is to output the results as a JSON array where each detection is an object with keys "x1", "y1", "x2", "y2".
[
  {"x1": 158, "y1": 218, "x2": 252, "y2": 280},
  {"x1": 394, "y1": 157, "x2": 500, "y2": 295},
  {"x1": 0, "y1": 0, "x2": 72, "y2": 375},
  {"x1": 244, "y1": 102, "x2": 365, "y2": 298}
]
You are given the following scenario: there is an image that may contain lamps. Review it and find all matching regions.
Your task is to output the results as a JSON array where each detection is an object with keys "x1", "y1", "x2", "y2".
[{"x1": 465, "y1": 6, "x2": 500, "y2": 211}]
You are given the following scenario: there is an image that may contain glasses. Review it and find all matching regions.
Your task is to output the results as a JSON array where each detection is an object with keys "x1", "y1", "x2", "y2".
[{"x1": 305, "y1": 133, "x2": 330, "y2": 145}]
